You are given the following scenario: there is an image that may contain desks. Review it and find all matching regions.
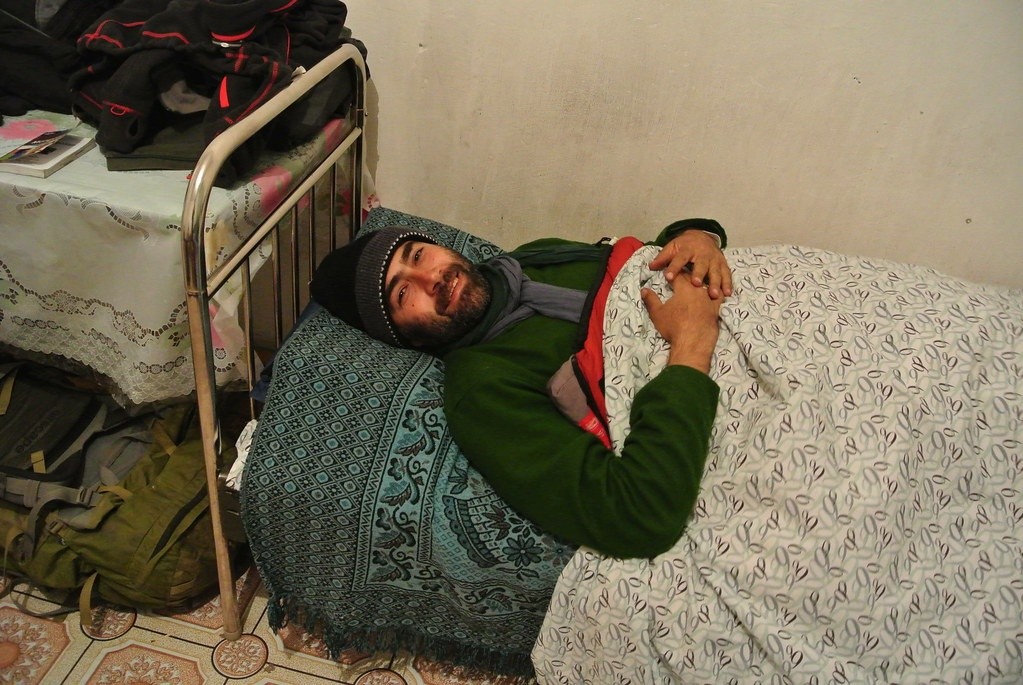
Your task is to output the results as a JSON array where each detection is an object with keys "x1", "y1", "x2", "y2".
[{"x1": 0, "y1": 99, "x2": 351, "y2": 420}]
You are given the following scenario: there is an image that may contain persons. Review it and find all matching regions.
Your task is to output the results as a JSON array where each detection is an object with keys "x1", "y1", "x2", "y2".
[{"x1": 308, "y1": 215, "x2": 735, "y2": 560}]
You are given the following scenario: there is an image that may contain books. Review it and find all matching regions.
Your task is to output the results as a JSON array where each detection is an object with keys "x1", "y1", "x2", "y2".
[{"x1": 0, "y1": 119, "x2": 96, "y2": 179}]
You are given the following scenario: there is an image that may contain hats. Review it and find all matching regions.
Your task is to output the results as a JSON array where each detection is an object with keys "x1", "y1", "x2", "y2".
[{"x1": 308, "y1": 226, "x2": 438, "y2": 349}]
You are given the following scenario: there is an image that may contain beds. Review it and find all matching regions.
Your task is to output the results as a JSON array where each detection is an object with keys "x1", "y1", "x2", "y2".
[{"x1": 200, "y1": 205, "x2": 1023, "y2": 685}]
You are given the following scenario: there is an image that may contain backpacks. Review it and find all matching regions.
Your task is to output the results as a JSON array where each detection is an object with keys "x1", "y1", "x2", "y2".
[{"x1": 2, "y1": 345, "x2": 256, "y2": 610}]
